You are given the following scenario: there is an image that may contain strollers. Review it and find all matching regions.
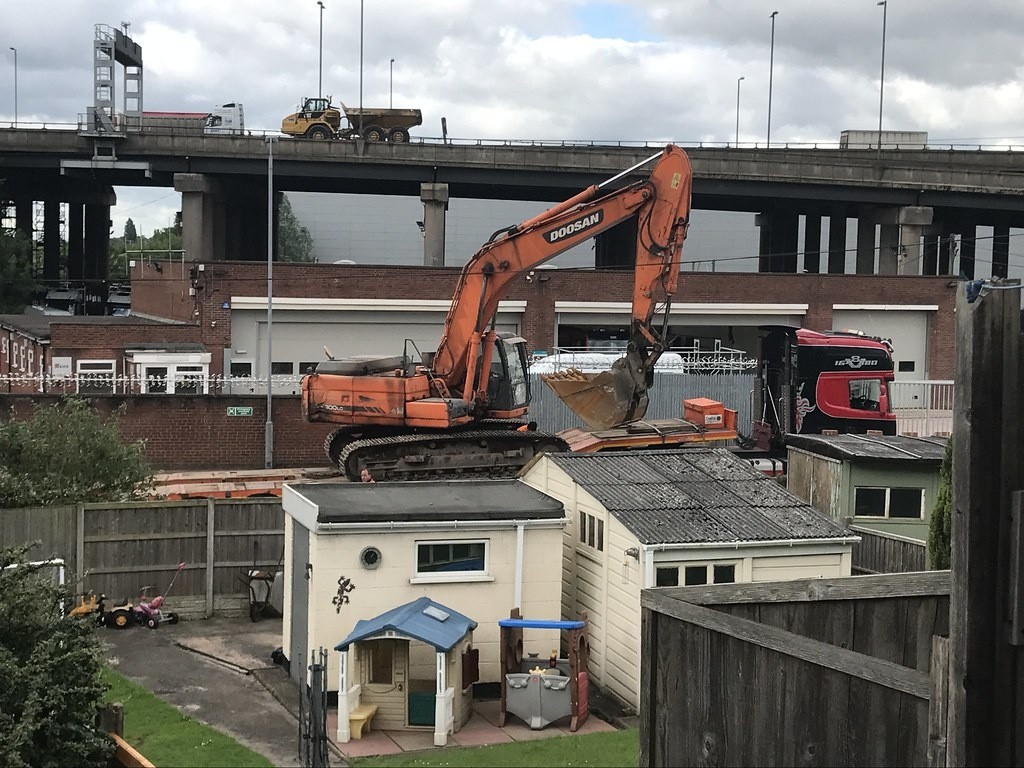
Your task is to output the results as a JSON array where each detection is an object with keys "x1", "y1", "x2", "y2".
[{"x1": 133, "y1": 560, "x2": 186, "y2": 630}]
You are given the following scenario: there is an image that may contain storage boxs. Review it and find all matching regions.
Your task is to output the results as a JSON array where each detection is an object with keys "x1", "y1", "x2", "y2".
[{"x1": 684, "y1": 397, "x2": 724, "y2": 430}]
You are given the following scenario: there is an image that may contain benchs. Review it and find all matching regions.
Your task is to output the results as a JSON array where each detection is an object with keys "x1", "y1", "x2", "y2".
[{"x1": 348, "y1": 705, "x2": 379, "y2": 740}]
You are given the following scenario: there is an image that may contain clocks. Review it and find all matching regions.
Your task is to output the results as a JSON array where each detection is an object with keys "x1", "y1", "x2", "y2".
[{"x1": 358, "y1": 546, "x2": 382, "y2": 569}]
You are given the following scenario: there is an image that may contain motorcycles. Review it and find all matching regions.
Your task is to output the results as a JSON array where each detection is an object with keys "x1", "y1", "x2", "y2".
[{"x1": 68, "y1": 588, "x2": 135, "y2": 631}]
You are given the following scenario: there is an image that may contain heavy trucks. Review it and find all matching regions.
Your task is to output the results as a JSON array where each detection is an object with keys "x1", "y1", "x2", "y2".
[
  {"x1": 279, "y1": 95, "x2": 425, "y2": 143},
  {"x1": 129, "y1": 321, "x2": 899, "y2": 499}
]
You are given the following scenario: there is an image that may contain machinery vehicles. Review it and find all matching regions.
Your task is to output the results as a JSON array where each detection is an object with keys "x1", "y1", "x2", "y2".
[{"x1": 299, "y1": 142, "x2": 695, "y2": 481}]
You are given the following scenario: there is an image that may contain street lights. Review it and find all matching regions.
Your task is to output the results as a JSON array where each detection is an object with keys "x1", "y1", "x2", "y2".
[
  {"x1": 316, "y1": 0, "x2": 325, "y2": 110},
  {"x1": 766, "y1": 10, "x2": 782, "y2": 149},
  {"x1": 260, "y1": 132, "x2": 281, "y2": 468},
  {"x1": 9, "y1": 46, "x2": 19, "y2": 129},
  {"x1": 389, "y1": 59, "x2": 397, "y2": 109},
  {"x1": 876, "y1": 0, "x2": 888, "y2": 165},
  {"x1": 736, "y1": 76, "x2": 745, "y2": 150}
]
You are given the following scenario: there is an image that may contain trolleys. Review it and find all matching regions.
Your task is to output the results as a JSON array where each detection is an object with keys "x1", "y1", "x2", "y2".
[{"x1": 237, "y1": 539, "x2": 284, "y2": 623}]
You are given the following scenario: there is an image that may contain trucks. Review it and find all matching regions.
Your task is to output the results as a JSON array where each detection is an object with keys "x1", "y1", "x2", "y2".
[{"x1": 203, "y1": 102, "x2": 295, "y2": 138}]
